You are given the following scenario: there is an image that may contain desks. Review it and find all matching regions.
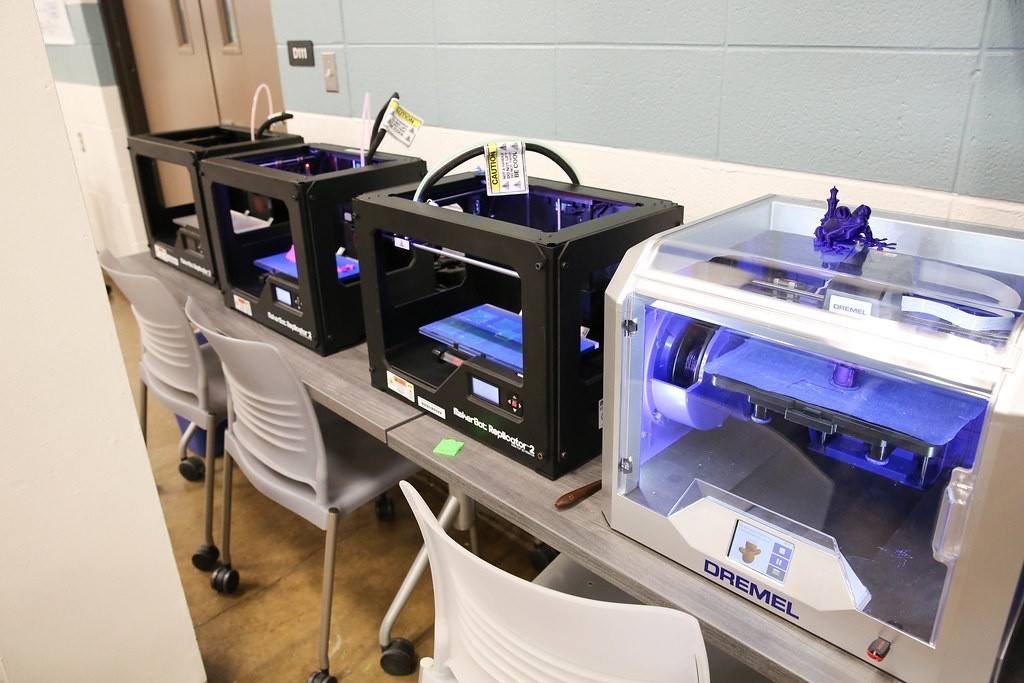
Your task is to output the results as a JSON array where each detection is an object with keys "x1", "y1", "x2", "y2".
[{"x1": 117, "y1": 252, "x2": 907, "y2": 683}]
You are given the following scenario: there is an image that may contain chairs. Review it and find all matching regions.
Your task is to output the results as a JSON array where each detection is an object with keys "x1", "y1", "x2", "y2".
[
  {"x1": 398, "y1": 478, "x2": 766, "y2": 683},
  {"x1": 98, "y1": 248, "x2": 227, "y2": 569},
  {"x1": 185, "y1": 295, "x2": 423, "y2": 683}
]
